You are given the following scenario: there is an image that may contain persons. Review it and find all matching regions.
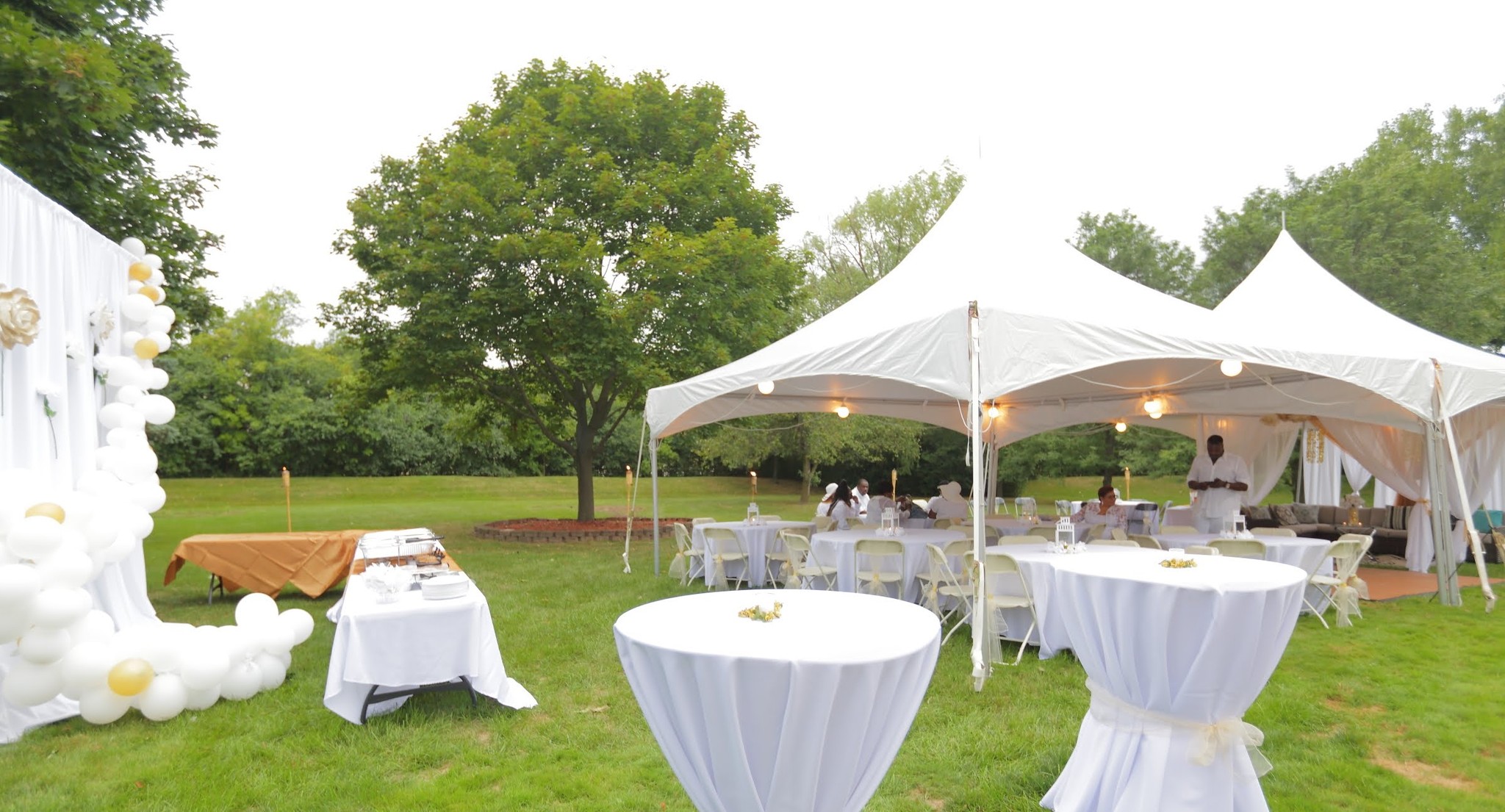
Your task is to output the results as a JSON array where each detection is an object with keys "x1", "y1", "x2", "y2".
[
  {"x1": 925, "y1": 481, "x2": 969, "y2": 522},
  {"x1": 849, "y1": 479, "x2": 871, "y2": 515},
  {"x1": 817, "y1": 483, "x2": 839, "y2": 517},
  {"x1": 1186, "y1": 434, "x2": 1250, "y2": 533},
  {"x1": 827, "y1": 483, "x2": 860, "y2": 531},
  {"x1": 866, "y1": 482, "x2": 912, "y2": 529},
  {"x1": 1049, "y1": 486, "x2": 1127, "y2": 540},
  {"x1": 1101, "y1": 474, "x2": 1120, "y2": 500}
]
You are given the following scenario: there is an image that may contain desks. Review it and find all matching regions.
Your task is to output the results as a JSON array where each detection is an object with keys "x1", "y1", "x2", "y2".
[
  {"x1": 1335, "y1": 526, "x2": 1379, "y2": 563},
  {"x1": 162, "y1": 529, "x2": 389, "y2": 606},
  {"x1": 1036, "y1": 549, "x2": 1307, "y2": 812},
  {"x1": 613, "y1": 589, "x2": 942, "y2": 812},
  {"x1": 689, "y1": 501, "x2": 1333, "y2": 647},
  {"x1": 322, "y1": 527, "x2": 538, "y2": 725}
]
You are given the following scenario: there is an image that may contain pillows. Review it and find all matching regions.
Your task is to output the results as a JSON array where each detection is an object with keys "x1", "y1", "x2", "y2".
[
  {"x1": 1472, "y1": 509, "x2": 1504, "y2": 533},
  {"x1": 1383, "y1": 505, "x2": 1411, "y2": 530},
  {"x1": 1250, "y1": 503, "x2": 1319, "y2": 525}
]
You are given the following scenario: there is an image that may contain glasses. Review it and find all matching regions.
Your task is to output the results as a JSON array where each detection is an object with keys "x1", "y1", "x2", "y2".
[{"x1": 1103, "y1": 495, "x2": 1118, "y2": 499}]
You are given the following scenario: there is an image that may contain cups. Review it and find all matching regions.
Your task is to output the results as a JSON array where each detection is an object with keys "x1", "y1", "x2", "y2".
[
  {"x1": 1169, "y1": 548, "x2": 1184, "y2": 561},
  {"x1": 1114, "y1": 491, "x2": 1119, "y2": 498}
]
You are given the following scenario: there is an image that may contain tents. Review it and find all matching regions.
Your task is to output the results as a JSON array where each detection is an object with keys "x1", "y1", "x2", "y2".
[
  {"x1": 1215, "y1": 212, "x2": 1505, "y2": 607},
  {"x1": 646, "y1": 175, "x2": 1447, "y2": 698}
]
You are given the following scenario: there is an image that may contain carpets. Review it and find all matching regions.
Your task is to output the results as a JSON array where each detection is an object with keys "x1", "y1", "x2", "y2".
[{"x1": 1331, "y1": 564, "x2": 1505, "y2": 601}]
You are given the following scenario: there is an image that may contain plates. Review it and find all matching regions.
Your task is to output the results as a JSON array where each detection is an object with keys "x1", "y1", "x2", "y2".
[{"x1": 421, "y1": 575, "x2": 470, "y2": 599}]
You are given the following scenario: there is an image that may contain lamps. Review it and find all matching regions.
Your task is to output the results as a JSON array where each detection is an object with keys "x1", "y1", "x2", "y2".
[
  {"x1": 837, "y1": 396, "x2": 849, "y2": 418},
  {"x1": 1220, "y1": 360, "x2": 1242, "y2": 376},
  {"x1": 988, "y1": 398, "x2": 998, "y2": 418},
  {"x1": 758, "y1": 380, "x2": 774, "y2": 394},
  {"x1": 1144, "y1": 390, "x2": 1160, "y2": 413},
  {"x1": 1149, "y1": 410, "x2": 1162, "y2": 419},
  {"x1": 1115, "y1": 418, "x2": 1126, "y2": 432}
]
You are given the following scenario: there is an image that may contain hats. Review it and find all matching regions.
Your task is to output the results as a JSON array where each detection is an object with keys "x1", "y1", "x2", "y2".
[
  {"x1": 822, "y1": 482, "x2": 838, "y2": 501},
  {"x1": 937, "y1": 480, "x2": 962, "y2": 501}
]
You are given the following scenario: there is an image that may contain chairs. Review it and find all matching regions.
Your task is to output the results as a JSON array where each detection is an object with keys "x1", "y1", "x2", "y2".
[
  {"x1": 1015, "y1": 496, "x2": 1038, "y2": 520},
  {"x1": 912, "y1": 499, "x2": 928, "y2": 510},
  {"x1": 1158, "y1": 499, "x2": 1173, "y2": 535},
  {"x1": 668, "y1": 515, "x2": 1373, "y2": 667},
  {"x1": 1071, "y1": 500, "x2": 1084, "y2": 515},
  {"x1": 986, "y1": 497, "x2": 1008, "y2": 515},
  {"x1": 1061, "y1": 500, "x2": 1075, "y2": 515},
  {"x1": 1055, "y1": 500, "x2": 1068, "y2": 515},
  {"x1": 970, "y1": 500, "x2": 987, "y2": 515}
]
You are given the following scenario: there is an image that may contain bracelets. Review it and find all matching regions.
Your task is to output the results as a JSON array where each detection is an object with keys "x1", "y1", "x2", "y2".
[{"x1": 1224, "y1": 481, "x2": 1230, "y2": 489}]
